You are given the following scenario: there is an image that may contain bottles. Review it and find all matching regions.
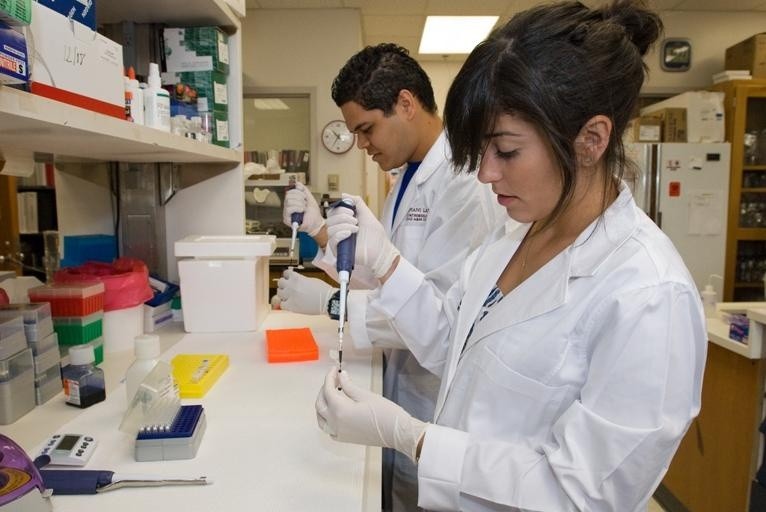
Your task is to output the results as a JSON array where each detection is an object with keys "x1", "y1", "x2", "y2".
[
  {"x1": 60, "y1": 343, "x2": 106, "y2": 410},
  {"x1": 121, "y1": 60, "x2": 213, "y2": 145},
  {"x1": 739, "y1": 127, "x2": 765, "y2": 288},
  {"x1": 189, "y1": 358, "x2": 210, "y2": 384},
  {"x1": 336, "y1": 361, "x2": 344, "y2": 393},
  {"x1": 123, "y1": 334, "x2": 172, "y2": 418},
  {"x1": 138, "y1": 395, "x2": 182, "y2": 436},
  {"x1": 288, "y1": 266, "x2": 294, "y2": 271},
  {"x1": 700, "y1": 283, "x2": 719, "y2": 318}
]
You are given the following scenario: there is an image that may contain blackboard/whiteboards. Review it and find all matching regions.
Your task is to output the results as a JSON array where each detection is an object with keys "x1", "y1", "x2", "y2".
[{"x1": 660, "y1": 142, "x2": 733, "y2": 303}]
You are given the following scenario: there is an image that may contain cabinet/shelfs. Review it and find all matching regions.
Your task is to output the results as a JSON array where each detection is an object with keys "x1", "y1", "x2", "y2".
[
  {"x1": 709, "y1": 80, "x2": 764, "y2": 302},
  {"x1": 0, "y1": 1, "x2": 244, "y2": 431}
]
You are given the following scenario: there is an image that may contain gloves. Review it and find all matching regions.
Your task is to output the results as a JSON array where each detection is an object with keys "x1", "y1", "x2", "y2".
[
  {"x1": 273, "y1": 266, "x2": 342, "y2": 321},
  {"x1": 322, "y1": 190, "x2": 404, "y2": 280},
  {"x1": 312, "y1": 362, "x2": 430, "y2": 465},
  {"x1": 278, "y1": 180, "x2": 325, "y2": 242}
]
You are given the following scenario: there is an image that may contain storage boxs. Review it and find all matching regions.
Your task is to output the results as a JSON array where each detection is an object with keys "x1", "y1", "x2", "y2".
[
  {"x1": 634, "y1": 107, "x2": 687, "y2": 144},
  {"x1": 724, "y1": 32, "x2": 765, "y2": 79}
]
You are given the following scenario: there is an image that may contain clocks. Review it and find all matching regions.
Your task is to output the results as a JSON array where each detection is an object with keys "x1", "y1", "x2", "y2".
[{"x1": 319, "y1": 118, "x2": 355, "y2": 155}]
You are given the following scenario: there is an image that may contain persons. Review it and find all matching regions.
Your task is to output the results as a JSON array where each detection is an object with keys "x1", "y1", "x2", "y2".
[
  {"x1": 314, "y1": 1, "x2": 710, "y2": 512},
  {"x1": 276, "y1": 43, "x2": 518, "y2": 511}
]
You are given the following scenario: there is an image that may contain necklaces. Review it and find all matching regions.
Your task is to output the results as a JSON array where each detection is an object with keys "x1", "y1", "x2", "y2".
[{"x1": 512, "y1": 221, "x2": 567, "y2": 287}]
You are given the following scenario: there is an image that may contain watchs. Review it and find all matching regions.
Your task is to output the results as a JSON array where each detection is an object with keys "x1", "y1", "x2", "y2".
[{"x1": 326, "y1": 289, "x2": 349, "y2": 322}]
